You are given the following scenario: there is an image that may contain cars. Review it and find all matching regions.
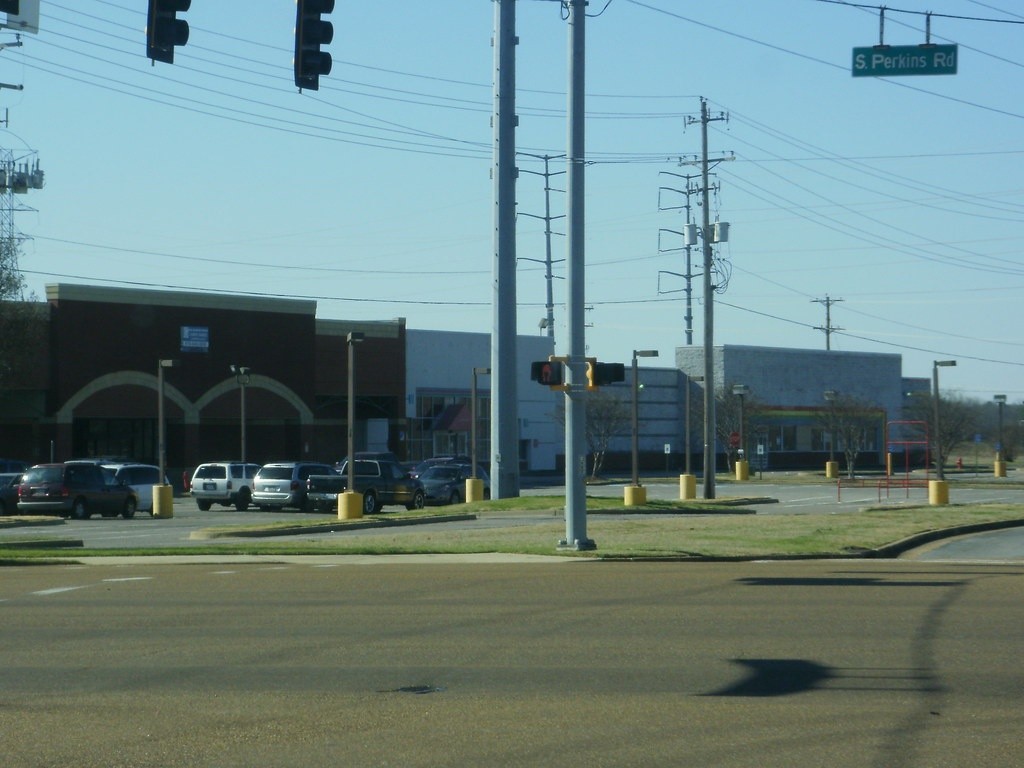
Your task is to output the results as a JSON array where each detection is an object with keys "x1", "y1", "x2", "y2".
[
  {"x1": 0, "y1": 473, "x2": 23, "y2": 516},
  {"x1": 335, "y1": 451, "x2": 398, "y2": 473},
  {"x1": 407, "y1": 456, "x2": 491, "y2": 507},
  {"x1": 17, "y1": 463, "x2": 139, "y2": 520}
]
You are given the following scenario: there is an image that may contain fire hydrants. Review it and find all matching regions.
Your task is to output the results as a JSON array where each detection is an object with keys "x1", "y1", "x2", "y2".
[
  {"x1": 957, "y1": 458, "x2": 963, "y2": 469},
  {"x1": 182, "y1": 471, "x2": 189, "y2": 491}
]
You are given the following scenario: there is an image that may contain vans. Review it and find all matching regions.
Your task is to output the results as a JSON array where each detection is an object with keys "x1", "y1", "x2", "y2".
[{"x1": 251, "y1": 462, "x2": 339, "y2": 511}]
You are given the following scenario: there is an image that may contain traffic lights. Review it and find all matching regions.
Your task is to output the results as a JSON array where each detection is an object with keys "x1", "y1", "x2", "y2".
[
  {"x1": 147, "y1": 0, "x2": 192, "y2": 65},
  {"x1": 594, "y1": 362, "x2": 626, "y2": 387},
  {"x1": 531, "y1": 362, "x2": 562, "y2": 385},
  {"x1": 294, "y1": 0, "x2": 335, "y2": 91}
]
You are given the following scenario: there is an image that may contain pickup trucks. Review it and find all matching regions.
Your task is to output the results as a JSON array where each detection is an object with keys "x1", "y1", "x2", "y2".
[{"x1": 306, "y1": 459, "x2": 426, "y2": 515}]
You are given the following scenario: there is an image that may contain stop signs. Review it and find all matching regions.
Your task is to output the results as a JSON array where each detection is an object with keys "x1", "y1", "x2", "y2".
[{"x1": 730, "y1": 433, "x2": 740, "y2": 445}]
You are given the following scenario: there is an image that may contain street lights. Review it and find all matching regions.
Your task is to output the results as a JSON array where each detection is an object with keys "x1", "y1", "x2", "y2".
[
  {"x1": 465, "y1": 368, "x2": 491, "y2": 503},
  {"x1": 680, "y1": 374, "x2": 704, "y2": 499},
  {"x1": 338, "y1": 331, "x2": 367, "y2": 520},
  {"x1": 152, "y1": 360, "x2": 178, "y2": 519},
  {"x1": 624, "y1": 349, "x2": 659, "y2": 506},
  {"x1": 994, "y1": 395, "x2": 1007, "y2": 477},
  {"x1": 733, "y1": 384, "x2": 750, "y2": 481},
  {"x1": 230, "y1": 366, "x2": 251, "y2": 463},
  {"x1": 928, "y1": 361, "x2": 957, "y2": 506}
]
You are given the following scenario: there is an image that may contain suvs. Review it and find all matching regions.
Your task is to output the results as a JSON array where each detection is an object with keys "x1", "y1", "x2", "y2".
[
  {"x1": 190, "y1": 461, "x2": 262, "y2": 511},
  {"x1": 64, "y1": 460, "x2": 171, "y2": 516}
]
